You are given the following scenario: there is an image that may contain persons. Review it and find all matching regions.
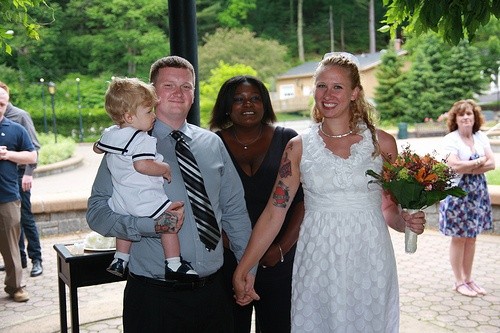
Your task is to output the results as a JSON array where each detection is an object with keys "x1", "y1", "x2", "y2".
[
  {"x1": 93, "y1": 76, "x2": 200, "y2": 284},
  {"x1": 433, "y1": 99, "x2": 496, "y2": 296},
  {"x1": 85, "y1": 55, "x2": 260, "y2": 333},
  {"x1": 0, "y1": 86, "x2": 38, "y2": 301},
  {"x1": 0, "y1": 79, "x2": 44, "y2": 276},
  {"x1": 233, "y1": 51, "x2": 428, "y2": 332},
  {"x1": 207, "y1": 76, "x2": 304, "y2": 333}
]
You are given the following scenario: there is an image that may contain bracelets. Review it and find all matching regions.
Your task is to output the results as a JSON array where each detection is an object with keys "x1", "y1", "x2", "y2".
[
  {"x1": 474, "y1": 158, "x2": 481, "y2": 169},
  {"x1": 276, "y1": 242, "x2": 283, "y2": 263}
]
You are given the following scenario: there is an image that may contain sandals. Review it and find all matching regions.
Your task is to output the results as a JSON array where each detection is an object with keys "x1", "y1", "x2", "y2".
[
  {"x1": 465, "y1": 279, "x2": 486, "y2": 295},
  {"x1": 455, "y1": 282, "x2": 477, "y2": 297}
]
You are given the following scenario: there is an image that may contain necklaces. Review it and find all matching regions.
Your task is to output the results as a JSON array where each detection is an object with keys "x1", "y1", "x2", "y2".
[
  {"x1": 232, "y1": 126, "x2": 263, "y2": 150},
  {"x1": 320, "y1": 122, "x2": 357, "y2": 138}
]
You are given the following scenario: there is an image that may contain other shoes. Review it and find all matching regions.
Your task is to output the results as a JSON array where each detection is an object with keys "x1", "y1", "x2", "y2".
[
  {"x1": 164, "y1": 260, "x2": 199, "y2": 280},
  {"x1": 107, "y1": 259, "x2": 127, "y2": 277},
  {"x1": 32, "y1": 260, "x2": 43, "y2": 277},
  {"x1": 0, "y1": 265, "x2": 7, "y2": 270},
  {"x1": 10, "y1": 291, "x2": 27, "y2": 302}
]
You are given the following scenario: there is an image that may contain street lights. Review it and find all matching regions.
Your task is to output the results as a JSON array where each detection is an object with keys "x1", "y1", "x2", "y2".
[{"x1": 48, "y1": 81, "x2": 58, "y2": 144}]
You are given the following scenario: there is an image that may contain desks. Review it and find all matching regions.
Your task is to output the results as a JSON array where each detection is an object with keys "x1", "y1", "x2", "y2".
[{"x1": 53, "y1": 241, "x2": 128, "y2": 333}]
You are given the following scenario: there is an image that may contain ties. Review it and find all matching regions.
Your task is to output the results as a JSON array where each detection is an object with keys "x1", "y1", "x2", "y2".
[{"x1": 168, "y1": 129, "x2": 222, "y2": 250}]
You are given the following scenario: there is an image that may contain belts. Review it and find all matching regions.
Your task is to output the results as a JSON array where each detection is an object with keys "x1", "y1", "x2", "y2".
[{"x1": 134, "y1": 274, "x2": 223, "y2": 290}]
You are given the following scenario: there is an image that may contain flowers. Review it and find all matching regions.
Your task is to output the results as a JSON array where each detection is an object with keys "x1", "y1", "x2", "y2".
[{"x1": 362, "y1": 141, "x2": 468, "y2": 255}]
